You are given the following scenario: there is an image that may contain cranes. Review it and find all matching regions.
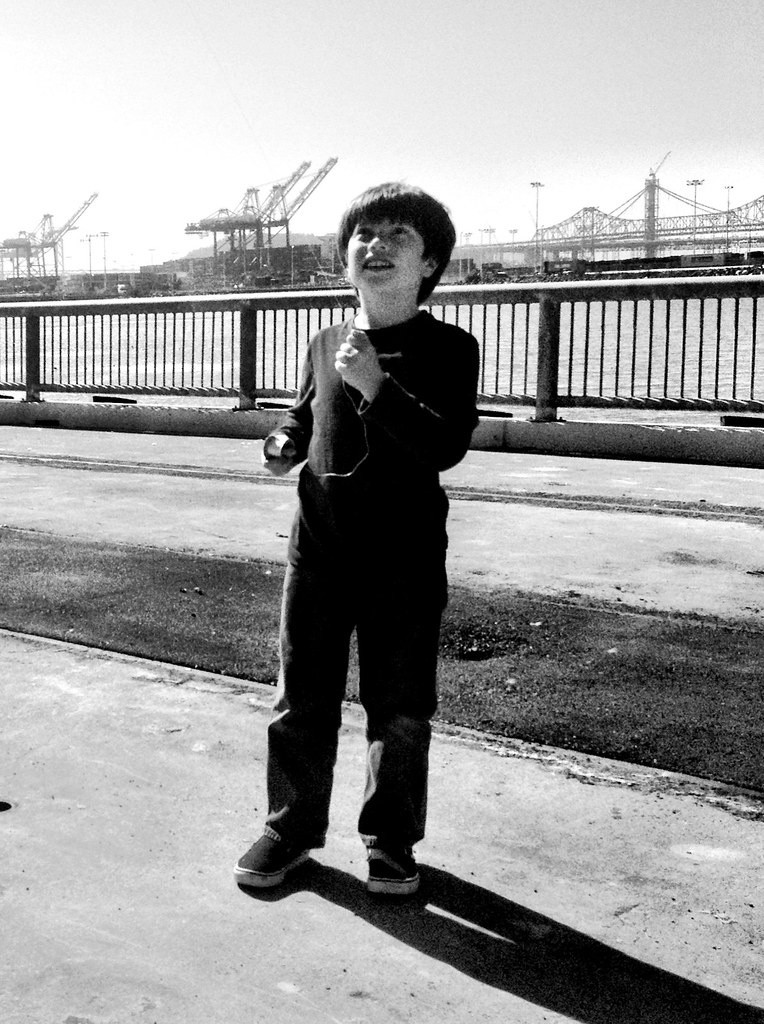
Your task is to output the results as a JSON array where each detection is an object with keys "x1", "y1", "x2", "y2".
[
  {"x1": 0, "y1": 193, "x2": 98, "y2": 293},
  {"x1": 184, "y1": 157, "x2": 339, "y2": 286}
]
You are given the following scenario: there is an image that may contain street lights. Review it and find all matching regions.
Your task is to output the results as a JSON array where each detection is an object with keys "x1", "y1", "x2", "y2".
[
  {"x1": 100, "y1": 231, "x2": 109, "y2": 288},
  {"x1": 724, "y1": 185, "x2": 733, "y2": 248},
  {"x1": 458, "y1": 228, "x2": 473, "y2": 279},
  {"x1": 80, "y1": 234, "x2": 98, "y2": 276},
  {"x1": 478, "y1": 228, "x2": 484, "y2": 277},
  {"x1": 530, "y1": 181, "x2": 545, "y2": 274},
  {"x1": 686, "y1": 179, "x2": 705, "y2": 255},
  {"x1": 509, "y1": 229, "x2": 517, "y2": 266}
]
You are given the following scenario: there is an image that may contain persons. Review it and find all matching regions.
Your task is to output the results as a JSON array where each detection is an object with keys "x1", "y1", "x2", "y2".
[{"x1": 232, "y1": 180, "x2": 480, "y2": 896}]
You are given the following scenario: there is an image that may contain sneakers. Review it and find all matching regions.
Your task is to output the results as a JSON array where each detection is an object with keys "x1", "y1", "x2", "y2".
[
  {"x1": 365, "y1": 846, "x2": 419, "y2": 894},
  {"x1": 233, "y1": 835, "x2": 310, "y2": 888}
]
